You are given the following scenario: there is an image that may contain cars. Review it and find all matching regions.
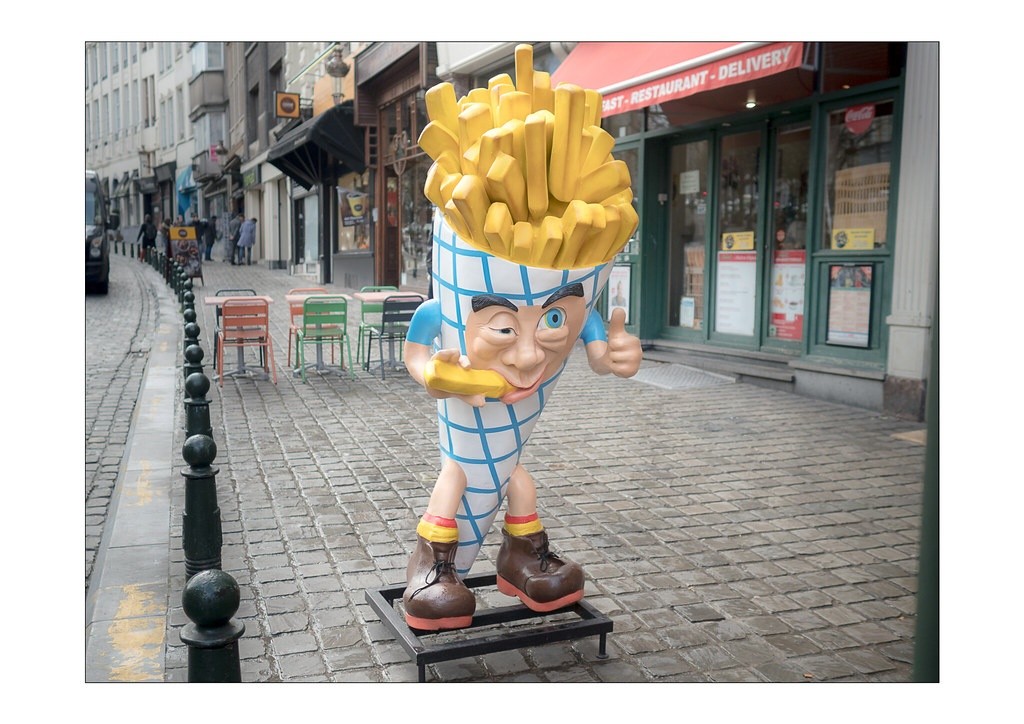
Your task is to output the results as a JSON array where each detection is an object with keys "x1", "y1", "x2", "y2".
[{"x1": 721, "y1": 188, "x2": 793, "y2": 222}]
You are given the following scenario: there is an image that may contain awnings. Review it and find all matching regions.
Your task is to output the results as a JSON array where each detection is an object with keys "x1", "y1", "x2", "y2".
[
  {"x1": 547, "y1": 40, "x2": 819, "y2": 121},
  {"x1": 267, "y1": 99, "x2": 374, "y2": 192}
]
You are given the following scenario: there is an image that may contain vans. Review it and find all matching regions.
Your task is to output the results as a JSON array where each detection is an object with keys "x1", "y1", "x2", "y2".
[
  {"x1": 685, "y1": 193, "x2": 698, "y2": 208},
  {"x1": 85, "y1": 170, "x2": 120, "y2": 295}
]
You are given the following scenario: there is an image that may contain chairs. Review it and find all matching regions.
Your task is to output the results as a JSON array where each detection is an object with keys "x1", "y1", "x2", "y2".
[{"x1": 212, "y1": 286, "x2": 424, "y2": 388}]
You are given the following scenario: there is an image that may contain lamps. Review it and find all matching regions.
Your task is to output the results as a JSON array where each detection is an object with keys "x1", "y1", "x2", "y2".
[
  {"x1": 743, "y1": 86, "x2": 758, "y2": 110},
  {"x1": 215, "y1": 139, "x2": 226, "y2": 170},
  {"x1": 324, "y1": 43, "x2": 349, "y2": 105}
]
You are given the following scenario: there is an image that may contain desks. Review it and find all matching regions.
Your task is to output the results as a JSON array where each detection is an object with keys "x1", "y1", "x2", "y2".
[
  {"x1": 353, "y1": 291, "x2": 429, "y2": 373},
  {"x1": 285, "y1": 294, "x2": 355, "y2": 378},
  {"x1": 203, "y1": 295, "x2": 276, "y2": 381}
]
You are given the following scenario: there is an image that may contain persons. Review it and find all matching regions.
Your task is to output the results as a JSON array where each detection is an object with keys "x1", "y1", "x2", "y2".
[
  {"x1": 173, "y1": 214, "x2": 185, "y2": 227},
  {"x1": 236, "y1": 218, "x2": 257, "y2": 265},
  {"x1": 203, "y1": 216, "x2": 217, "y2": 260},
  {"x1": 188, "y1": 215, "x2": 203, "y2": 263},
  {"x1": 401, "y1": 44, "x2": 643, "y2": 628},
  {"x1": 156, "y1": 218, "x2": 170, "y2": 262},
  {"x1": 136, "y1": 214, "x2": 159, "y2": 264},
  {"x1": 228, "y1": 214, "x2": 245, "y2": 266}
]
